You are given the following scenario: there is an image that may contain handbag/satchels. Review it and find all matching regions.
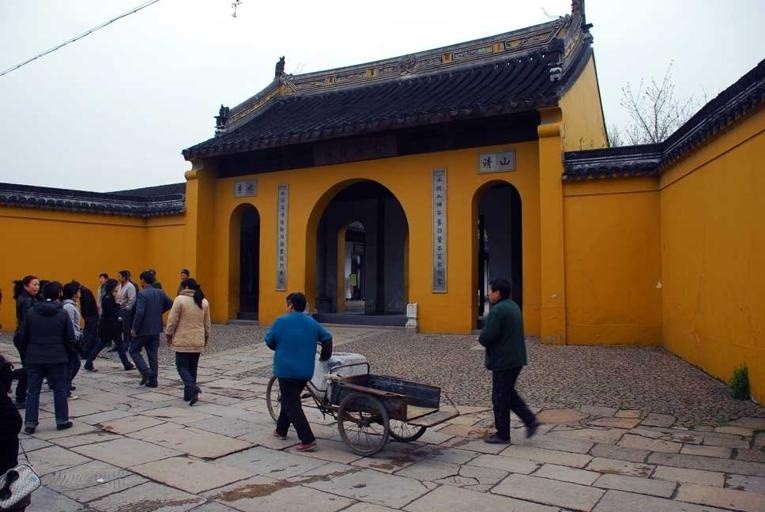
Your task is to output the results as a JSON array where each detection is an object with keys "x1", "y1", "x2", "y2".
[{"x1": 0, "y1": 463, "x2": 40, "y2": 510}]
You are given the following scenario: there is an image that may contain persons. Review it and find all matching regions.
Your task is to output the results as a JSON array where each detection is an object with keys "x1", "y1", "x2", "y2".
[
  {"x1": 6, "y1": 269, "x2": 188, "y2": 433},
  {"x1": 478, "y1": 279, "x2": 538, "y2": 446},
  {"x1": 165, "y1": 278, "x2": 212, "y2": 406},
  {"x1": 1, "y1": 356, "x2": 22, "y2": 474},
  {"x1": 262, "y1": 292, "x2": 333, "y2": 453}
]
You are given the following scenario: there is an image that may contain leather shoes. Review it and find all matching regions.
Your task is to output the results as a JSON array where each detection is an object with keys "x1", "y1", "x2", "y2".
[{"x1": 15, "y1": 365, "x2": 202, "y2": 433}]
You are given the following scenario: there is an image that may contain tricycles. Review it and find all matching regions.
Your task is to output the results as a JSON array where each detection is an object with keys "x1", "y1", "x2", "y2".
[{"x1": 266, "y1": 342, "x2": 459, "y2": 457}]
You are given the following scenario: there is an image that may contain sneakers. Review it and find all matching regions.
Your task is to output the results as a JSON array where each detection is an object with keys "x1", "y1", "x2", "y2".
[
  {"x1": 485, "y1": 434, "x2": 511, "y2": 444},
  {"x1": 526, "y1": 421, "x2": 540, "y2": 439},
  {"x1": 273, "y1": 429, "x2": 285, "y2": 440},
  {"x1": 297, "y1": 441, "x2": 317, "y2": 451}
]
